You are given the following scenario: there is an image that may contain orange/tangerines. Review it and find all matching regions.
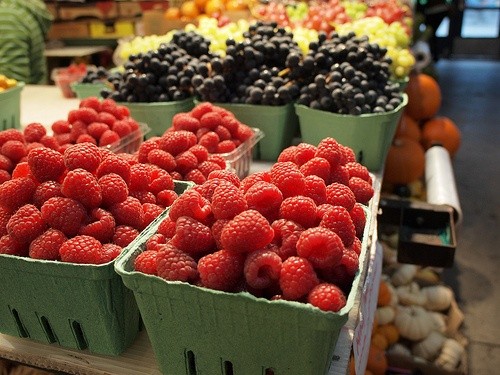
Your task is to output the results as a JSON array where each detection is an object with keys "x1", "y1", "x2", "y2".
[{"x1": 162, "y1": 0, "x2": 257, "y2": 20}]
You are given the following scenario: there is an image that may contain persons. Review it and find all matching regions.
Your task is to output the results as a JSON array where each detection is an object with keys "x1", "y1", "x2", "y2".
[{"x1": 0, "y1": 0, "x2": 55, "y2": 84}]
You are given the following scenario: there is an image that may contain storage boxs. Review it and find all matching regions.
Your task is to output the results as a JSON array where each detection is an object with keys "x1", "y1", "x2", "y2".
[
  {"x1": 44, "y1": 0, "x2": 143, "y2": 45},
  {"x1": 396, "y1": 206, "x2": 458, "y2": 269},
  {"x1": 102, "y1": 120, "x2": 151, "y2": 153},
  {"x1": 70, "y1": 83, "x2": 106, "y2": 100},
  {"x1": 110, "y1": 98, "x2": 195, "y2": 141},
  {"x1": 215, "y1": 121, "x2": 266, "y2": 174},
  {"x1": 0, "y1": 176, "x2": 196, "y2": 357},
  {"x1": 195, "y1": 98, "x2": 289, "y2": 161},
  {"x1": 0, "y1": 80, "x2": 28, "y2": 131},
  {"x1": 289, "y1": 88, "x2": 411, "y2": 167},
  {"x1": 50, "y1": 68, "x2": 79, "y2": 99},
  {"x1": 112, "y1": 185, "x2": 377, "y2": 375}
]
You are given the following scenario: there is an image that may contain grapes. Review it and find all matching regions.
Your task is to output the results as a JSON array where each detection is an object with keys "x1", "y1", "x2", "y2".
[
  {"x1": 195, "y1": 0, "x2": 415, "y2": 117},
  {"x1": 78, "y1": 23, "x2": 222, "y2": 103}
]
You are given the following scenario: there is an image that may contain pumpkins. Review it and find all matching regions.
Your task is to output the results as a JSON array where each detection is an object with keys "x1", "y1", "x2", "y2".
[
  {"x1": 348, "y1": 263, "x2": 464, "y2": 375},
  {"x1": 384, "y1": 71, "x2": 459, "y2": 191}
]
